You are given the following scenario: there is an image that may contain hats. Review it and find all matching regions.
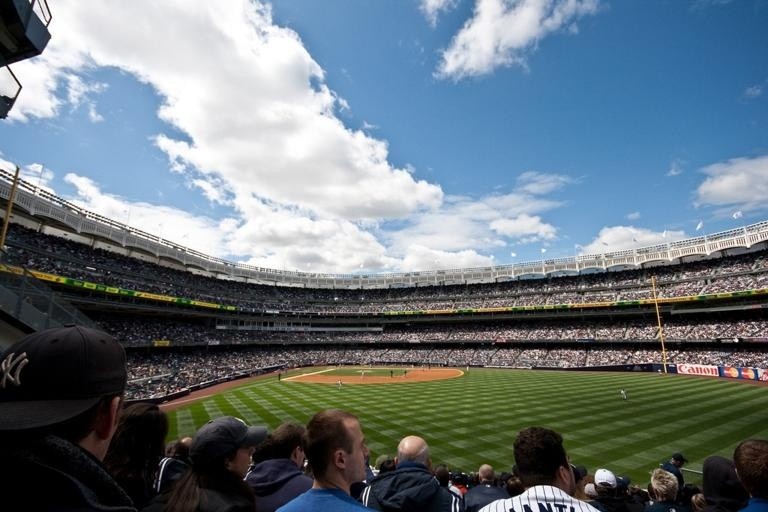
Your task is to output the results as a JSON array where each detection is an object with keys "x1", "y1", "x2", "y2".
[
  {"x1": 569, "y1": 463, "x2": 587, "y2": 481},
  {"x1": 594, "y1": 468, "x2": 616, "y2": 489},
  {"x1": 1, "y1": 323, "x2": 128, "y2": 430},
  {"x1": 672, "y1": 453, "x2": 688, "y2": 463},
  {"x1": 190, "y1": 415, "x2": 268, "y2": 457},
  {"x1": 617, "y1": 475, "x2": 631, "y2": 489}
]
